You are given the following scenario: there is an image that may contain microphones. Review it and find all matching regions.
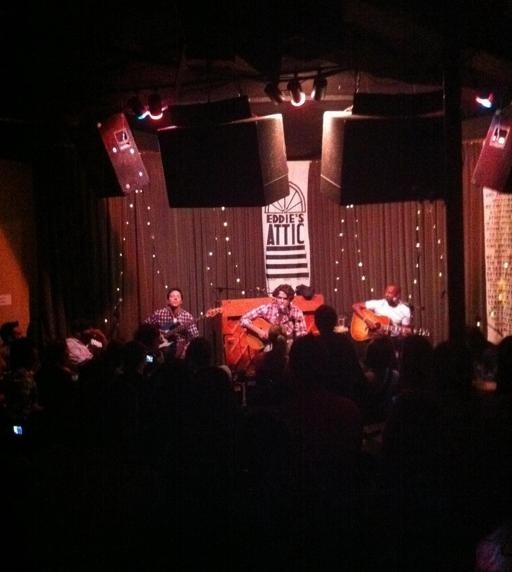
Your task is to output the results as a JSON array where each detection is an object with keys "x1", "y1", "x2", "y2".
[{"x1": 278, "y1": 304, "x2": 287, "y2": 314}]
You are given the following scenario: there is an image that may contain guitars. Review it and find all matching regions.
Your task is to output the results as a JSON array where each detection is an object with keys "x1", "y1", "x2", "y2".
[
  {"x1": 350, "y1": 308, "x2": 433, "y2": 343},
  {"x1": 246, "y1": 318, "x2": 313, "y2": 351},
  {"x1": 158, "y1": 306, "x2": 224, "y2": 348}
]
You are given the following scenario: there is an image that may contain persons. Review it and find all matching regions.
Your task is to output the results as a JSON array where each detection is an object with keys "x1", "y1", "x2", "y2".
[
  {"x1": 239, "y1": 284, "x2": 308, "y2": 366},
  {"x1": 1, "y1": 305, "x2": 511, "y2": 431},
  {"x1": 145, "y1": 289, "x2": 200, "y2": 358},
  {"x1": 352, "y1": 284, "x2": 412, "y2": 367}
]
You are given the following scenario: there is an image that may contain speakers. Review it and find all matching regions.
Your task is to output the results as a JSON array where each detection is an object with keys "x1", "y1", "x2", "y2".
[
  {"x1": 471, "y1": 111, "x2": 512, "y2": 192},
  {"x1": 78, "y1": 111, "x2": 151, "y2": 198},
  {"x1": 319, "y1": 92, "x2": 452, "y2": 205},
  {"x1": 156, "y1": 96, "x2": 291, "y2": 208}
]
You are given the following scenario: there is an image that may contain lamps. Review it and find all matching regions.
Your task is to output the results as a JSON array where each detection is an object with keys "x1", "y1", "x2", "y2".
[
  {"x1": 127, "y1": 94, "x2": 147, "y2": 119},
  {"x1": 477, "y1": 85, "x2": 497, "y2": 109},
  {"x1": 147, "y1": 93, "x2": 162, "y2": 119},
  {"x1": 312, "y1": 74, "x2": 328, "y2": 102},
  {"x1": 288, "y1": 78, "x2": 306, "y2": 106},
  {"x1": 265, "y1": 80, "x2": 285, "y2": 104}
]
